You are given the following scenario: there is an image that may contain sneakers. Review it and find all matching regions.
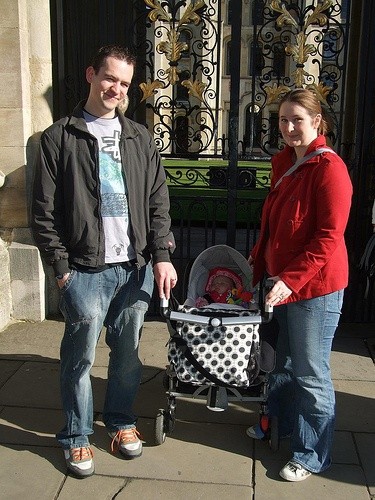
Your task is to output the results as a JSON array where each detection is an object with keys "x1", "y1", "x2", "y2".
[
  {"x1": 107, "y1": 422, "x2": 143, "y2": 459},
  {"x1": 63, "y1": 446, "x2": 95, "y2": 477},
  {"x1": 279, "y1": 460, "x2": 313, "y2": 482},
  {"x1": 247, "y1": 421, "x2": 291, "y2": 438}
]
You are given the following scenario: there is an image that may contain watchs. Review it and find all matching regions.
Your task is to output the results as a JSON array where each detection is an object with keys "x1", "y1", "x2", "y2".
[{"x1": 56, "y1": 272, "x2": 67, "y2": 279}]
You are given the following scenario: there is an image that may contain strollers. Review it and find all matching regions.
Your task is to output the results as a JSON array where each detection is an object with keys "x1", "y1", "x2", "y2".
[{"x1": 155, "y1": 243, "x2": 282, "y2": 451}]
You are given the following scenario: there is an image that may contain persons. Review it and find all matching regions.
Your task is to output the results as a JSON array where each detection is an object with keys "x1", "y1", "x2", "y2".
[
  {"x1": 246, "y1": 88, "x2": 352, "y2": 482},
  {"x1": 198, "y1": 267, "x2": 251, "y2": 309},
  {"x1": 31, "y1": 44, "x2": 178, "y2": 477}
]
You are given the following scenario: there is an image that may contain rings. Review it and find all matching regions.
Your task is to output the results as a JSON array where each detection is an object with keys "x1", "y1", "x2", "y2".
[
  {"x1": 170, "y1": 278, "x2": 176, "y2": 281},
  {"x1": 279, "y1": 294, "x2": 284, "y2": 299}
]
